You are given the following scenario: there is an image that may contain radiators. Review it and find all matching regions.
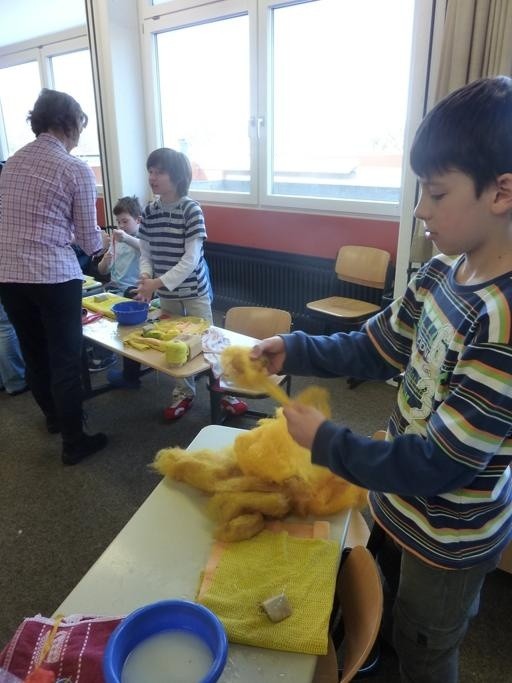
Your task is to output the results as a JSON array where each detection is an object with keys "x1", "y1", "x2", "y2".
[{"x1": 202, "y1": 241, "x2": 395, "y2": 336}]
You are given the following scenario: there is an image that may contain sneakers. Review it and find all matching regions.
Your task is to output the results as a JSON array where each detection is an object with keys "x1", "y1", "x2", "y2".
[
  {"x1": 216, "y1": 395, "x2": 249, "y2": 416},
  {"x1": 88, "y1": 353, "x2": 118, "y2": 372},
  {"x1": 163, "y1": 389, "x2": 195, "y2": 420}
]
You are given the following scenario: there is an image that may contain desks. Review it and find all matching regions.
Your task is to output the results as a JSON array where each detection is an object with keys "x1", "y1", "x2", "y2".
[
  {"x1": 82, "y1": 293, "x2": 265, "y2": 425},
  {"x1": 46, "y1": 424, "x2": 354, "y2": 683}
]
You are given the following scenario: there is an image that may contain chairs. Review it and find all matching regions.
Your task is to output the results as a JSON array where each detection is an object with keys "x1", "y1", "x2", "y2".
[
  {"x1": 372, "y1": 430, "x2": 387, "y2": 442},
  {"x1": 308, "y1": 544, "x2": 385, "y2": 683},
  {"x1": 206, "y1": 306, "x2": 290, "y2": 425},
  {"x1": 306, "y1": 245, "x2": 391, "y2": 390}
]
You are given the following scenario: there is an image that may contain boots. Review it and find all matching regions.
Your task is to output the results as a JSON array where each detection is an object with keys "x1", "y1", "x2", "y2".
[{"x1": 36, "y1": 401, "x2": 108, "y2": 465}]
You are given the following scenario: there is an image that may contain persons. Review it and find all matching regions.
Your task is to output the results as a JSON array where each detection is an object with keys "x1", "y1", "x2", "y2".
[
  {"x1": 134, "y1": 148, "x2": 249, "y2": 420},
  {"x1": 88, "y1": 197, "x2": 140, "y2": 371},
  {"x1": 0, "y1": 87, "x2": 110, "y2": 467},
  {"x1": 237, "y1": 78, "x2": 512, "y2": 683}
]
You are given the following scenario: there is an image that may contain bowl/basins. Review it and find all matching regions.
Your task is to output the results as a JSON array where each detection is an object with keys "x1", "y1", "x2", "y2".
[
  {"x1": 110, "y1": 300, "x2": 149, "y2": 325},
  {"x1": 102, "y1": 599, "x2": 229, "y2": 682}
]
